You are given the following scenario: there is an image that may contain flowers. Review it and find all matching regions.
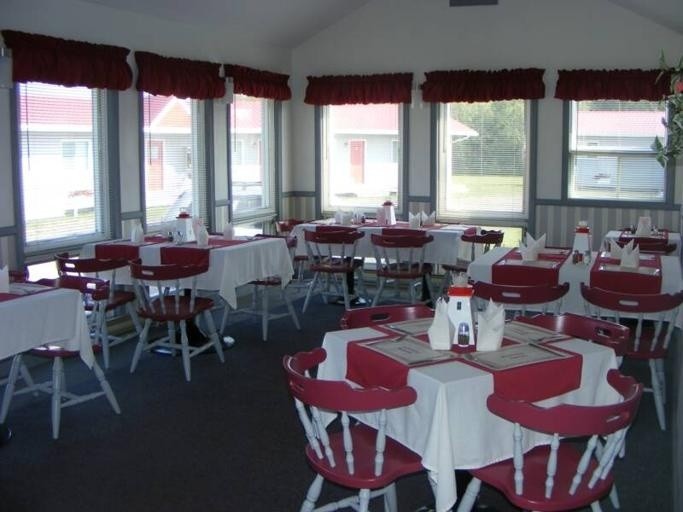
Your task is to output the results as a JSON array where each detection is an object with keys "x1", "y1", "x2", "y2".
[{"x1": 651, "y1": 46, "x2": 683, "y2": 168}]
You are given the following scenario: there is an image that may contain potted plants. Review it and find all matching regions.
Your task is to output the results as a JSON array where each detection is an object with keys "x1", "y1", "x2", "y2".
[{"x1": 594, "y1": 174, "x2": 611, "y2": 186}]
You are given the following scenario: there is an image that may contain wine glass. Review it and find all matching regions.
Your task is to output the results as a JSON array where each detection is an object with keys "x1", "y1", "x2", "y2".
[{"x1": 604, "y1": 240, "x2": 609, "y2": 256}]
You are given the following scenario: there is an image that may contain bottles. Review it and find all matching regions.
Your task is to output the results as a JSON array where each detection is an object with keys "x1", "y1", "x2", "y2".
[
  {"x1": 572, "y1": 250, "x2": 591, "y2": 266},
  {"x1": 167, "y1": 231, "x2": 184, "y2": 245},
  {"x1": 458, "y1": 324, "x2": 470, "y2": 347}
]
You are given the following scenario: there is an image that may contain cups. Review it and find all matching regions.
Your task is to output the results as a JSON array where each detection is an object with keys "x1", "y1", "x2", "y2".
[
  {"x1": 658, "y1": 232, "x2": 662, "y2": 239},
  {"x1": 451, "y1": 272, "x2": 468, "y2": 287},
  {"x1": 579, "y1": 220, "x2": 587, "y2": 226}
]
